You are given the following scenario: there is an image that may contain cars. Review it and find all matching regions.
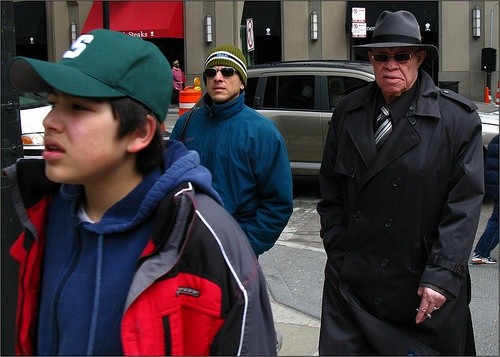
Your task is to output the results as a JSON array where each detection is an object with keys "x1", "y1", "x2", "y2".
[{"x1": 20, "y1": 91, "x2": 56, "y2": 160}]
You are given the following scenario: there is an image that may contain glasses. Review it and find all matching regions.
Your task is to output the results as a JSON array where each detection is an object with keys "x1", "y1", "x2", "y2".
[
  {"x1": 204, "y1": 67, "x2": 236, "y2": 78},
  {"x1": 370, "y1": 49, "x2": 418, "y2": 62}
]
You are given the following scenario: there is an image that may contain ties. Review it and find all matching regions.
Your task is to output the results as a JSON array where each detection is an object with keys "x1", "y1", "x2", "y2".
[{"x1": 374, "y1": 104, "x2": 392, "y2": 149}]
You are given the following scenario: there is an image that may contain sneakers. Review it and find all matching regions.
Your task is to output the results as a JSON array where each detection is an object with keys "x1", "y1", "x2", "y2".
[{"x1": 471, "y1": 251, "x2": 497, "y2": 264}]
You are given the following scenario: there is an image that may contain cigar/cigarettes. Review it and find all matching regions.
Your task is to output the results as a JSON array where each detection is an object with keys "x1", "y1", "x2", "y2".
[{"x1": 415, "y1": 308, "x2": 432, "y2": 320}]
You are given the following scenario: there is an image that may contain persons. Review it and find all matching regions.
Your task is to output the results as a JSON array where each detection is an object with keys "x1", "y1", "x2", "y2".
[
  {"x1": 317, "y1": 7, "x2": 484, "y2": 357},
  {"x1": 167, "y1": 42, "x2": 294, "y2": 357},
  {"x1": 1, "y1": 27, "x2": 279, "y2": 357},
  {"x1": 171, "y1": 60, "x2": 184, "y2": 107},
  {"x1": 470, "y1": 132, "x2": 500, "y2": 265}
]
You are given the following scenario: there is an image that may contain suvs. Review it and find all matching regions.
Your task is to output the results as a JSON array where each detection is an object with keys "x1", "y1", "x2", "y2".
[{"x1": 246, "y1": 59, "x2": 500, "y2": 185}]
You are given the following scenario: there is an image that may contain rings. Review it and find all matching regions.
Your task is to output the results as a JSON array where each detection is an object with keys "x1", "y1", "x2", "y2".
[{"x1": 434, "y1": 306, "x2": 439, "y2": 311}]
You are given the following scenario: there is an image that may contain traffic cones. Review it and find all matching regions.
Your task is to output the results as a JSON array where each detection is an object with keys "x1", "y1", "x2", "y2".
[{"x1": 483, "y1": 86, "x2": 491, "y2": 104}]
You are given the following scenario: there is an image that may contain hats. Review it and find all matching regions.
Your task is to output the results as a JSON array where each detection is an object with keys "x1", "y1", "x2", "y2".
[
  {"x1": 351, "y1": 10, "x2": 439, "y2": 61},
  {"x1": 202, "y1": 44, "x2": 248, "y2": 87},
  {"x1": 9, "y1": 28, "x2": 173, "y2": 124}
]
